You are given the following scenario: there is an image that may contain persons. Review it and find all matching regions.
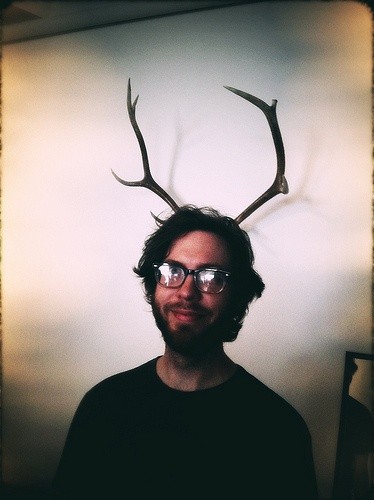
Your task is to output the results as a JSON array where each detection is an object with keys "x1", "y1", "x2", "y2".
[{"x1": 48, "y1": 205, "x2": 317, "y2": 499}]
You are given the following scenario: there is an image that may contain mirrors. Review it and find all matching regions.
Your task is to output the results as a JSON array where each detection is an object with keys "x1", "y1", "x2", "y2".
[{"x1": 333, "y1": 351, "x2": 374, "y2": 499}]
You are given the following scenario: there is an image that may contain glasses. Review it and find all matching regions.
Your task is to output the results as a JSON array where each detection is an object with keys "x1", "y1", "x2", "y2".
[{"x1": 152, "y1": 259, "x2": 235, "y2": 294}]
